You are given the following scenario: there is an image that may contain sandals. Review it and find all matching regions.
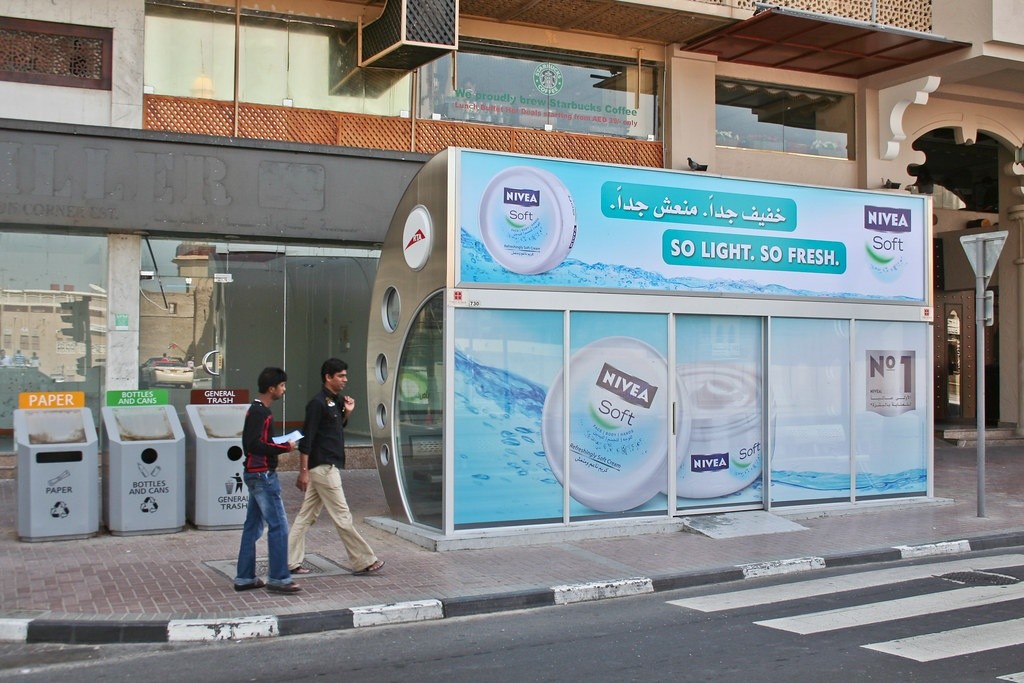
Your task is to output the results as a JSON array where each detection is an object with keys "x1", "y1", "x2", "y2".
[
  {"x1": 265, "y1": 582, "x2": 304, "y2": 592},
  {"x1": 233, "y1": 576, "x2": 269, "y2": 591}
]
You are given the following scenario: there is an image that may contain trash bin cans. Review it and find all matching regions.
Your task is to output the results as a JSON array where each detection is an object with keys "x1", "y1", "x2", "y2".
[
  {"x1": 12, "y1": 409, "x2": 100, "y2": 543},
  {"x1": 184, "y1": 404, "x2": 251, "y2": 530},
  {"x1": 101, "y1": 406, "x2": 186, "y2": 537}
]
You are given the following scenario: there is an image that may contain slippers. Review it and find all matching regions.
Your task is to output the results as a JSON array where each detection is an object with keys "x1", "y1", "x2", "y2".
[
  {"x1": 353, "y1": 560, "x2": 386, "y2": 575},
  {"x1": 290, "y1": 566, "x2": 310, "y2": 574}
]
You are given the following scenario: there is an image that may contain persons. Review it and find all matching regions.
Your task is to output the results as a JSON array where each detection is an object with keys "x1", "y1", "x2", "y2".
[
  {"x1": 14, "y1": 350, "x2": 25, "y2": 364},
  {"x1": 0, "y1": 349, "x2": 13, "y2": 365},
  {"x1": 161, "y1": 353, "x2": 169, "y2": 362},
  {"x1": 29, "y1": 352, "x2": 41, "y2": 366},
  {"x1": 288, "y1": 358, "x2": 384, "y2": 574},
  {"x1": 234, "y1": 367, "x2": 302, "y2": 592},
  {"x1": 187, "y1": 355, "x2": 195, "y2": 368}
]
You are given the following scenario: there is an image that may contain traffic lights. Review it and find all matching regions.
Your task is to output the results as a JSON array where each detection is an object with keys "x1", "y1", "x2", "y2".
[
  {"x1": 76, "y1": 357, "x2": 84, "y2": 375},
  {"x1": 60, "y1": 301, "x2": 84, "y2": 343}
]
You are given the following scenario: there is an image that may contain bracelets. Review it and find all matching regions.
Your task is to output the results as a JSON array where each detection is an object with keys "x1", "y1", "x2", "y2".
[{"x1": 302, "y1": 468, "x2": 306, "y2": 470}]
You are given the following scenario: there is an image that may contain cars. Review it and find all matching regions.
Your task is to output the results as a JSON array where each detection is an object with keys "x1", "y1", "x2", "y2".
[{"x1": 139, "y1": 356, "x2": 194, "y2": 388}]
